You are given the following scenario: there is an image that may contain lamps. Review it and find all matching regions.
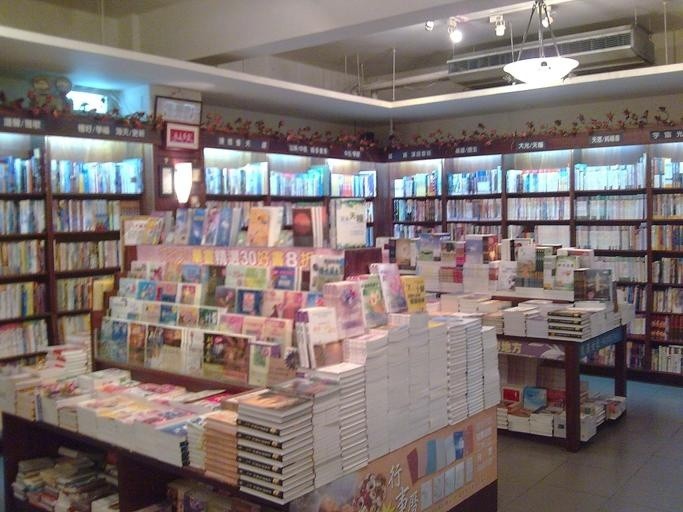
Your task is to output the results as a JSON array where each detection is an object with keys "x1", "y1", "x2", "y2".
[
  {"x1": 502, "y1": 0, "x2": 579, "y2": 85},
  {"x1": 489, "y1": 15, "x2": 506, "y2": 37},
  {"x1": 448, "y1": 21, "x2": 462, "y2": 43},
  {"x1": 537, "y1": 6, "x2": 554, "y2": 28}
]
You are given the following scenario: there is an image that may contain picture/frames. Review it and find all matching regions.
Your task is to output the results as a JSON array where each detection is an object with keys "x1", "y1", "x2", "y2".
[
  {"x1": 159, "y1": 164, "x2": 174, "y2": 198},
  {"x1": 163, "y1": 121, "x2": 202, "y2": 152},
  {"x1": 154, "y1": 93, "x2": 203, "y2": 127}
]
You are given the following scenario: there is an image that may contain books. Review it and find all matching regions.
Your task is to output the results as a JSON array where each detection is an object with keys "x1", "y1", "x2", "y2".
[{"x1": 1, "y1": 146, "x2": 682, "y2": 510}]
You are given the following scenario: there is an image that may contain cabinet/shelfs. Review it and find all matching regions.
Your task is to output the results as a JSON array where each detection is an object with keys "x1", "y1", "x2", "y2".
[
  {"x1": 426, "y1": 290, "x2": 628, "y2": 452},
  {"x1": 649, "y1": 141, "x2": 683, "y2": 387},
  {"x1": 48, "y1": 129, "x2": 156, "y2": 346},
  {"x1": 503, "y1": 148, "x2": 572, "y2": 248},
  {"x1": 0, "y1": 247, "x2": 497, "y2": 512},
  {"x1": 203, "y1": 145, "x2": 268, "y2": 230},
  {"x1": 0, "y1": 131, "x2": 53, "y2": 370},
  {"x1": 268, "y1": 150, "x2": 330, "y2": 232},
  {"x1": 443, "y1": 153, "x2": 503, "y2": 238},
  {"x1": 330, "y1": 158, "x2": 389, "y2": 277},
  {"x1": 572, "y1": 143, "x2": 649, "y2": 383},
  {"x1": 389, "y1": 156, "x2": 443, "y2": 239}
]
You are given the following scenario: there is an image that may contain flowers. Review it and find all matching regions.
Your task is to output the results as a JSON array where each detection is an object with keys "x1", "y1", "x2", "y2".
[
  {"x1": 0, "y1": 89, "x2": 165, "y2": 128},
  {"x1": 391, "y1": 106, "x2": 683, "y2": 151},
  {"x1": 204, "y1": 113, "x2": 391, "y2": 157}
]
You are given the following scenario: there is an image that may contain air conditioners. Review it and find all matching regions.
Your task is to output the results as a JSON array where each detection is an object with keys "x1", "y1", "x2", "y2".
[{"x1": 447, "y1": 13, "x2": 655, "y2": 90}]
